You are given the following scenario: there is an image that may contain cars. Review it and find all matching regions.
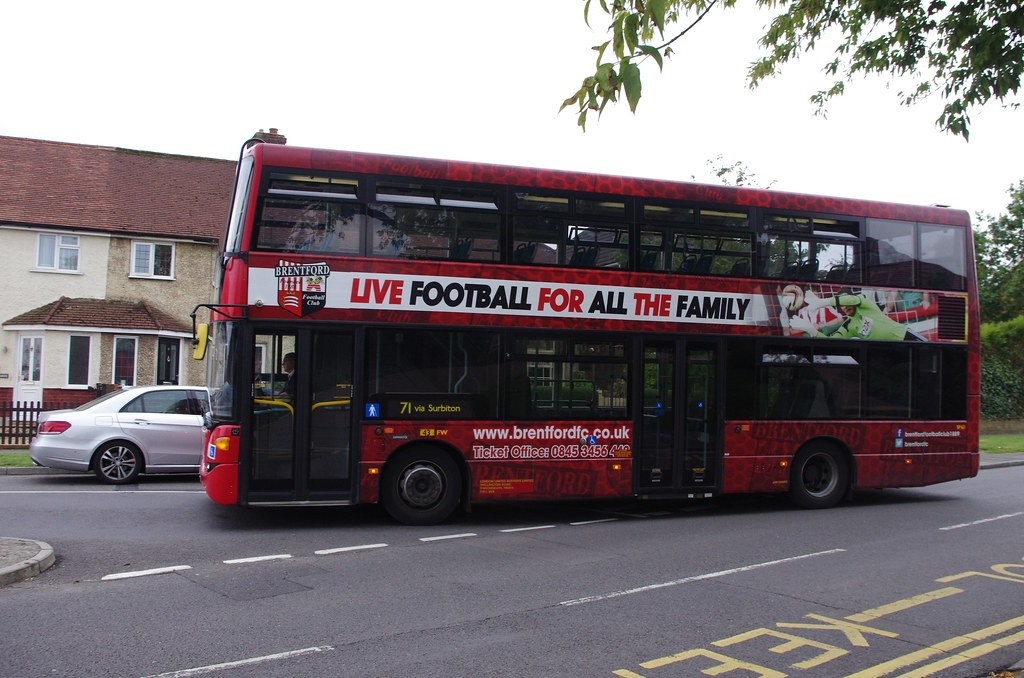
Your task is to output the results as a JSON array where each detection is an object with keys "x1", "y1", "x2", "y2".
[{"x1": 28, "y1": 386, "x2": 209, "y2": 484}]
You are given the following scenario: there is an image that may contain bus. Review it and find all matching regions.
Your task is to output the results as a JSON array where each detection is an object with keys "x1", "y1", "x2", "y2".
[{"x1": 188, "y1": 136, "x2": 986, "y2": 526}]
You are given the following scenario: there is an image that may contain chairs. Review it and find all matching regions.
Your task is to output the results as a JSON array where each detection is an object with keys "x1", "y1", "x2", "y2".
[{"x1": 300, "y1": 225, "x2": 862, "y2": 286}]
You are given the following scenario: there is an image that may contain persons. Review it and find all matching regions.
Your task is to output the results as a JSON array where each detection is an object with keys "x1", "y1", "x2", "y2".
[
  {"x1": 269, "y1": 353, "x2": 325, "y2": 406},
  {"x1": 789, "y1": 287, "x2": 929, "y2": 342}
]
